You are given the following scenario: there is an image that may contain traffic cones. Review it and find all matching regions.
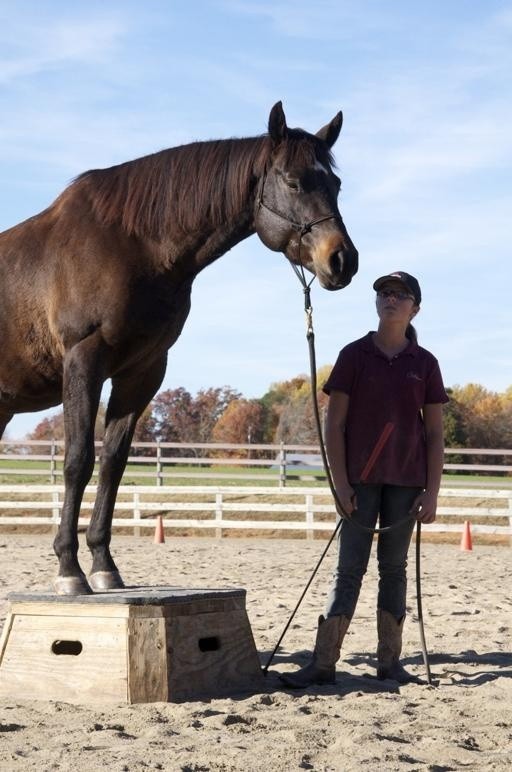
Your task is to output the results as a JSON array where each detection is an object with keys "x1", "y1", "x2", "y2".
[
  {"x1": 458, "y1": 520, "x2": 474, "y2": 552},
  {"x1": 153, "y1": 515, "x2": 166, "y2": 543}
]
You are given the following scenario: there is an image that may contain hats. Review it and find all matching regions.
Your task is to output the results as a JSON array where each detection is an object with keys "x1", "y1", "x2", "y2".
[{"x1": 373, "y1": 272, "x2": 421, "y2": 303}]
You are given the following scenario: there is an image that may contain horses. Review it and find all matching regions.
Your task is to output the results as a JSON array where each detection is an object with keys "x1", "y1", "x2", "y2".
[{"x1": 0, "y1": 97, "x2": 360, "y2": 599}]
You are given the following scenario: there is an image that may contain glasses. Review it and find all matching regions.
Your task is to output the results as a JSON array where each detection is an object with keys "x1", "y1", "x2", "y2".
[{"x1": 377, "y1": 289, "x2": 415, "y2": 303}]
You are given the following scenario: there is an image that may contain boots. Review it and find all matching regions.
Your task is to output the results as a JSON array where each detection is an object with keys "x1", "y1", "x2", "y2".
[
  {"x1": 377, "y1": 608, "x2": 423, "y2": 685},
  {"x1": 281, "y1": 615, "x2": 351, "y2": 687}
]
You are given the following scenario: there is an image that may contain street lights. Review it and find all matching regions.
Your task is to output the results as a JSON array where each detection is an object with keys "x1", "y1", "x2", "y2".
[{"x1": 247, "y1": 426, "x2": 253, "y2": 460}]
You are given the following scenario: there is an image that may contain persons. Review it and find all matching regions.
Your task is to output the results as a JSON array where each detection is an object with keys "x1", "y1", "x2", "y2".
[{"x1": 278, "y1": 271, "x2": 449, "y2": 688}]
